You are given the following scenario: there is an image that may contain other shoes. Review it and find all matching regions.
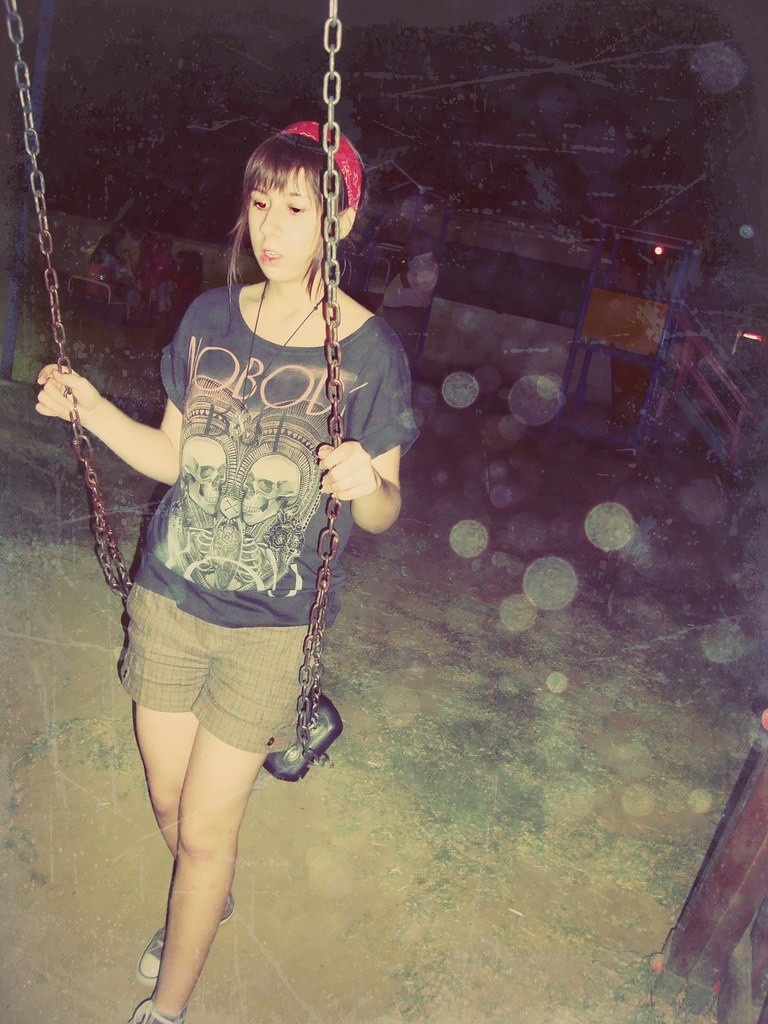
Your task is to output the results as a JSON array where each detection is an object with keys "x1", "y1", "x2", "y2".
[
  {"x1": 128, "y1": 994, "x2": 188, "y2": 1024},
  {"x1": 136, "y1": 891, "x2": 235, "y2": 986}
]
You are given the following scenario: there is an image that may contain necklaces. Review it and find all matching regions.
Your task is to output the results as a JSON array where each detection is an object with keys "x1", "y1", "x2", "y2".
[{"x1": 228, "y1": 280, "x2": 326, "y2": 437}]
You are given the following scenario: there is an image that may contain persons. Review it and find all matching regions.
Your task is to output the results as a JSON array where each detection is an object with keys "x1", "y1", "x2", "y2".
[
  {"x1": 382, "y1": 217, "x2": 437, "y2": 358},
  {"x1": 36, "y1": 123, "x2": 421, "y2": 1024},
  {"x1": 88, "y1": 227, "x2": 203, "y2": 325}
]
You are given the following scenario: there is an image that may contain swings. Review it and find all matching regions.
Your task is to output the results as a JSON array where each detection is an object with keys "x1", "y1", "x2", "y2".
[{"x1": 6, "y1": 0, "x2": 350, "y2": 782}]
